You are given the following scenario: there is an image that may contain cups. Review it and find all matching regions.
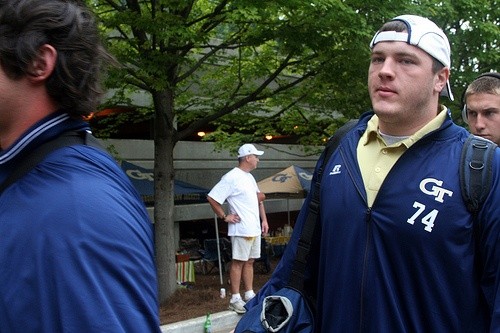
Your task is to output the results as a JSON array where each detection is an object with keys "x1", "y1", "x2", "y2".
[{"x1": 220, "y1": 288, "x2": 226, "y2": 298}]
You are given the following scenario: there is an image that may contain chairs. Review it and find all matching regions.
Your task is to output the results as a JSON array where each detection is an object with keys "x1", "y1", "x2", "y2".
[{"x1": 198, "y1": 237, "x2": 272, "y2": 275}]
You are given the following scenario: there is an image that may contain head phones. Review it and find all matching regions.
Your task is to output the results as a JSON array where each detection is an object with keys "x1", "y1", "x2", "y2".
[{"x1": 460, "y1": 72, "x2": 500, "y2": 125}]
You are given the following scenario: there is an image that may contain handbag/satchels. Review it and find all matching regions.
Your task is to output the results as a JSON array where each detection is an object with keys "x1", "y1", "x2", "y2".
[{"x1": 232, "y1": 287, "x2": 316, "y2": 333}]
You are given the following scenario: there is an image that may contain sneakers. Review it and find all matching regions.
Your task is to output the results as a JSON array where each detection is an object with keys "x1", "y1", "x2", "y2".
[
  {"x1": 244, "y1": 294, "x2": 256, "y2": 301},
  {"x1": 229, "y1": 298, "x2": 247, "y2": 313}
]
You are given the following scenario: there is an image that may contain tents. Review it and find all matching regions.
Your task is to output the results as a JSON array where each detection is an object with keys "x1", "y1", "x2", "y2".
[
  {"x1": 116, "y1": 158, "x2": 226, "y2": 299},
  {"x1": 257, "y1": 164, "x2": 313, "y2": 227}
]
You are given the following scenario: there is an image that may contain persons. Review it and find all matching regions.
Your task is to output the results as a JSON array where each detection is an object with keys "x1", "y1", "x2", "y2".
[
  {"x1": 233, "y1": 15, "x2": 500, "y2": 333},
  {"x1": 206, "y1": 143, "x2": 269, "y2": 313},
  {"x1": 0, "y1": 0, "x2": 164, "y2": 333}
]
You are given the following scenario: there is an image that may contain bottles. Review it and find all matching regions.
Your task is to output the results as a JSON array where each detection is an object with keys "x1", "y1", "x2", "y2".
[{"x1": 203, "y1": 313, "x2": 211, "y2": 333}]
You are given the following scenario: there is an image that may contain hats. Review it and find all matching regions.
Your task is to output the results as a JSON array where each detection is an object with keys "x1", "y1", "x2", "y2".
[
  {"x1": 237, "y1": 143, "x2": 264, "y2": 159},
  {"x1": 369, "y1": 14, "x2": 455, "y2": 101}
]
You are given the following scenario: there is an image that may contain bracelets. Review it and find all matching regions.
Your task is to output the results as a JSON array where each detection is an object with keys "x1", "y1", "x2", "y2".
[{"x1": 220, "y1": 214, "x2": 228, "y2": 222}]
